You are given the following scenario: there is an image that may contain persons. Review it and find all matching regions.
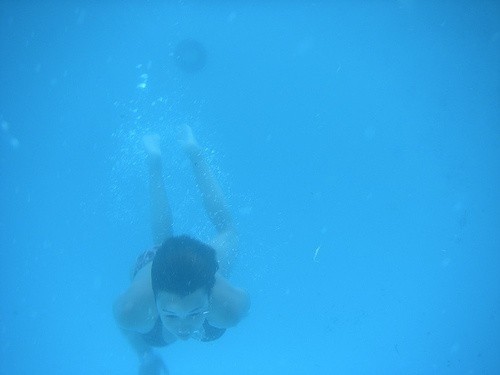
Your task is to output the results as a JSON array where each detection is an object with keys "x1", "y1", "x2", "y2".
[{"x1": 112, "y1": 122, "x2": 255, "y2": 375}]
